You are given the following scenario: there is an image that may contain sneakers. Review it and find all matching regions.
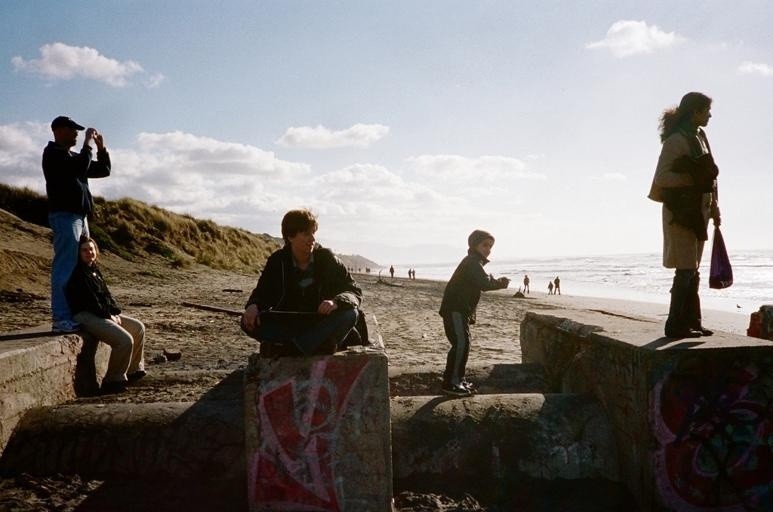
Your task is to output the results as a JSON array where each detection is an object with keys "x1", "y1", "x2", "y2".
[
  {"x1": 458, "y1": 377, "x2": 477, "y2": 394},
  {"x1": 52, "y1": 320, "x2": 81, "y2": 334},
  {"x1": 442, "y1": 382, "x2": 471, "y2": 397},
  {"x1": 67, "y1": 318, "x2": 81, "y2": 327}
]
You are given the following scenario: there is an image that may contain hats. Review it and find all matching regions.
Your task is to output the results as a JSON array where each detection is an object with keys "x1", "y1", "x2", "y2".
[{"x1": 469, "y1": 230, "x2": 495, "y2": 250}]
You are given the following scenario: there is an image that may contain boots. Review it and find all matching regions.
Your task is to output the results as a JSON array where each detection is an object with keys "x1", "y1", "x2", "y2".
[{"x1": 51, "y1": 116, "x2": 84, "y2": 130}]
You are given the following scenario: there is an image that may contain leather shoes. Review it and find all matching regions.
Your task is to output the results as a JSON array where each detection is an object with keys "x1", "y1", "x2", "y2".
[
  {"x1": 667, "y1": 328, "x2": 703, "y2": 339},
  {"x1": 698, "y1": 325, "x2": 713, "y2": 337}
]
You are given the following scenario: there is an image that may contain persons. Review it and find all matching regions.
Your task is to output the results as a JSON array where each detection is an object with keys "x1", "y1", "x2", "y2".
[
  {"x1": 347, "y1": 265, "x2": 372, "y2": 272},
  {"x1": 546, "y1": 281, "x2": 554, "y2": 296},
  {"x1": 407, "y1": 269, "x2": 412, "y2": 278},
  {"x1": 65, "y1": 233, "x2": 147, "y2": 390},
  {"x1": 238, "y1": 209, "x2": 365, "y2": 353},
  {"x1": 436, "y1": 228, "x2": 510, "y2": 397},
  {"x1": 42, "y1": 115, "x2": 111, "y2": 335},
  {"x1": 647, "y1": 90, "x2": 723, "y2": 337},
  {"x1": 553, "y1": 275, "x2": 562, "y2": 296},
  {"x1": 521, "y1": 274, "x2": 531, "y2": 293},
  {"x1": 390, "y1": 265, "x2": 396, "y2": 278},
  {"x1": 411, "y1": 269, "x2": 415, "y2": 280}
]
